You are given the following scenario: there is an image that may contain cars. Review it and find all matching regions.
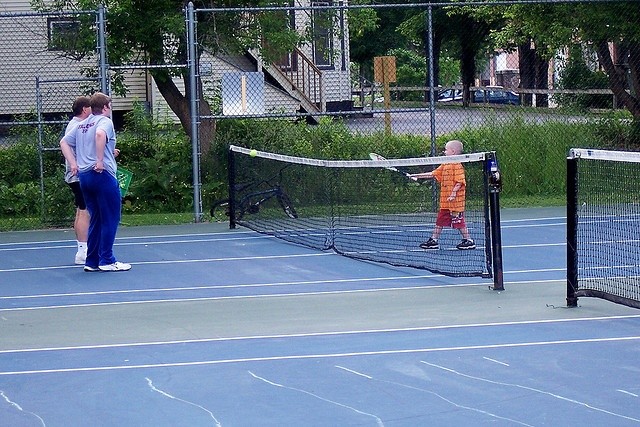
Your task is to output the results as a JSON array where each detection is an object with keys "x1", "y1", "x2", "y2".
[
  {"x1": 438, "y1": 87, "x2": 521, "y2": 106},
  {"x1": 437, "y1": 85, "x2": 469, "y2": 101}
]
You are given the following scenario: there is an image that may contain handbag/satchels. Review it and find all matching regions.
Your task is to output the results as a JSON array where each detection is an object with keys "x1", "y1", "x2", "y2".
[{"x1": 116, "y1": 165, "x2": 133, "y2": 198}]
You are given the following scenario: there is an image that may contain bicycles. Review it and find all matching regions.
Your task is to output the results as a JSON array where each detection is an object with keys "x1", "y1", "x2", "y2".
[{"x1": 210, "y1": 162, "x2": 298, "y2": 221}]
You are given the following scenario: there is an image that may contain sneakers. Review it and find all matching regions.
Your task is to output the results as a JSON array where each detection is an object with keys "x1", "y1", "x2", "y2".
[
  {"x1": 455, "y1": 237, "x2": 477, "y2": 251},
  {"x1": 418, "y1": 236, "x2": 441, "y2": 251},
  {"x1": 83, "y1": 265, "x2": 102, "y2": 273},
  {"x1": 97, "y1": 260, "x2": 132, "y2": 272},
  {"x1": 74, "y1": 247, "x2": 88, "y2": 265}
]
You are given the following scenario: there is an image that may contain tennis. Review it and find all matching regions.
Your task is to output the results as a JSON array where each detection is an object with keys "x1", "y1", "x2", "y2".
[{"x1": 249, "y1": 150, "x2": 257, "y2": 157}]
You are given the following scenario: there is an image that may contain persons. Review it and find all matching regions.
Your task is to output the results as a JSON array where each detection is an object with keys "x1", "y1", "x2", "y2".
[
  {"x1": 411, "y1": 139, "x2": 476, "y2": 249},
  {"x1": 65, "y1": 97, "x2": 119, "y2": 264},
  {"x1": 60, "y1": 92, "x2": 132, "y2": 271}
]
